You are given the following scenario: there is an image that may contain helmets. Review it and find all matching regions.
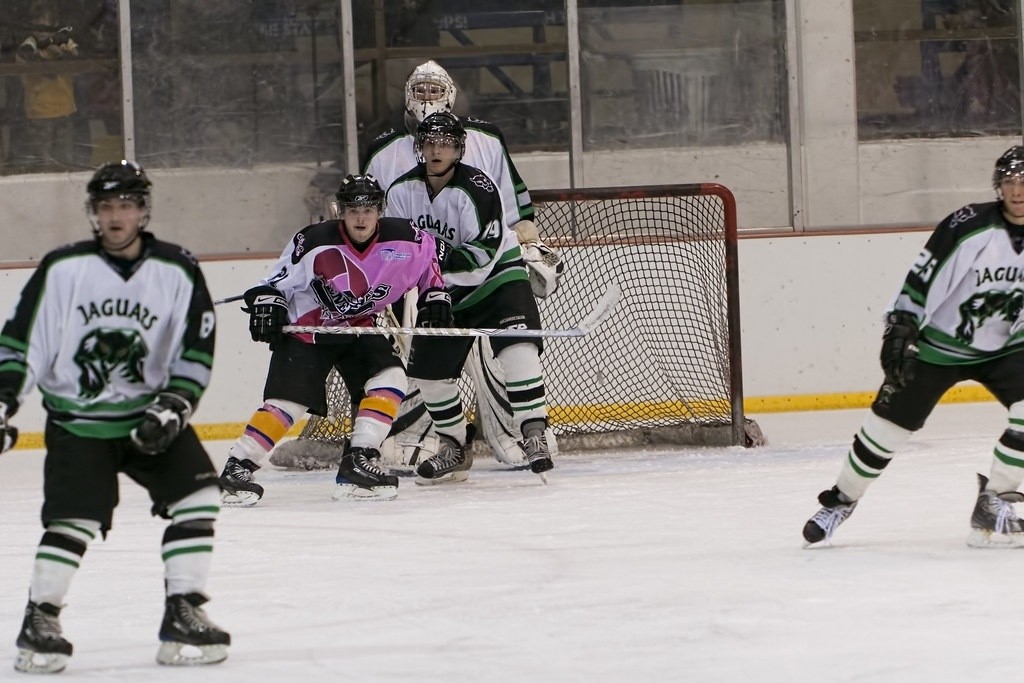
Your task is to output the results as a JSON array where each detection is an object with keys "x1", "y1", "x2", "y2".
[
  {"x1": 994, "y1": 145, "x2": 1024, "y2": 171},
  {"x1": 403, "y1": 61, "x2": 456, "y2": 122},
  {"x1": 416, "y1": 111, "x2": 465, "y2": 144},
  {"x1": 335, "y1": 173, "x2": 385, "y2": 207},
  {"x1": 86, "y1": 160, "x2": 152, "y2": 208}
]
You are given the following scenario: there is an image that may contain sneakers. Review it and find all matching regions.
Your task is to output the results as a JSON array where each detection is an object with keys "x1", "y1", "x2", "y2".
[
  {"x1": 801, "y1": 487, "x2": 858, "y2": 548},
  {"x1": 331, "y1": 433, "x2": 399, "y2": 501},
  {"x1": 15, "y1": 591, "x2": 73, "y2": 673},
  {"x1": 157, "y1": 582, "x2": 228, "y2": 666},
  {"x1": 518, "y1": 417, "x2": 556, "y2": 484},
  {"x1": 217, "y1": 455, "x2": 264, "y2": 508},
  {"x1": 414, "y1": 422, "x2": 477, "y2": 486},
  {"x1": 966, "y1": 472, "x2": 1024, "y2": 548}
]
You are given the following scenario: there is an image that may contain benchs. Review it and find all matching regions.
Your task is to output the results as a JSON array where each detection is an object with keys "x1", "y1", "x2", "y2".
[
  {"x1": 771, "y1": 0, "x2": 1023, "y2": 137},
  {"x1": 0, "y1": 0, "x2": 643, "y2": 150}
]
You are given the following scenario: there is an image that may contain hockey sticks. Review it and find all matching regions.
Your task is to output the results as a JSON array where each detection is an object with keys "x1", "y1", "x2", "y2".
[
  {"x1": 210, "y1": 293, "x2": 245, "y2": 306},
  {"x1": 282, "y1": 284, "x2": 622, "y2": 337}
]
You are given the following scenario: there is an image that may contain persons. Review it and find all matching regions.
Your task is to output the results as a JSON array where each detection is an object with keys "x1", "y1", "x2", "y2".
[
  {"x1": 218, "y1": 173, "x2": 453, "y2": 507},
  {"x1": 801, "y1": 145, "x2": 1024, "y2": 550},
  {"x1": 0, "y1": 161, "x2": 231, "y2": 683},
  {"x1": 364, "y1": 60, "x2": 554, "y2": 509},
  {"x1": 18, "y1": 3, "x2": 80, "y2": 177}
]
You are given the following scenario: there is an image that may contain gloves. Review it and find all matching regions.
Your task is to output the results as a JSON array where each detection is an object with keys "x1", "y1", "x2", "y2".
[
  {"x1": 248, "y1": 293, "x2": 287, "y2": 351},
  {"x1": 0, "y1": 392, "x2": 20, "y2": 453},
  {"x1": 880, "y1": 308, "x2": 919, "y2": 388},
  {"x1": 416, "y1": 290, "x2": 454, "y2": 331},
  {"x1": 129, "y1": 386, "x2": 198, "y2": 455},
  {"x1": 435, "y1": 234, "x2": 454, "y2": 268}
]
